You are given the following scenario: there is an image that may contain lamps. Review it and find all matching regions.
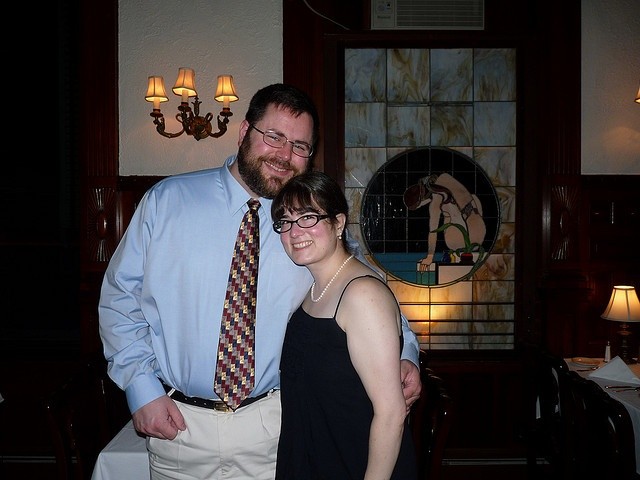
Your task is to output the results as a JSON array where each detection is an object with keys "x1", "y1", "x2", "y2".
[
  {"x1": 601, "y1": 285, "x2": 640, "y2": 364},
  {"x1": 145, "y1": 68, "x2": 239, "y2": 141}
]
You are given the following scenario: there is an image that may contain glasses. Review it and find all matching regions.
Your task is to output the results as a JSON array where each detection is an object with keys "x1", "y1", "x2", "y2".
[
  {"x1": 250, "y1": 125, "x2": 315, "y2": 157},
  {"x1": 272, "y1": 214, "x2": 333, "y2": 233}
]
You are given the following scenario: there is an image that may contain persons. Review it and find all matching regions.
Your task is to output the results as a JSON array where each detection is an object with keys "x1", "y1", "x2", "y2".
[
  {"x1": 274, "y1": 172, "x2": 409, "y2": 480},
  {"x1": 98, "y1": 84, "x2": 423, "y2": 480}
]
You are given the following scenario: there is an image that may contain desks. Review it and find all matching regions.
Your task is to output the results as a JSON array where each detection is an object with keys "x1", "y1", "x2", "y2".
[{"x1": 559, "y1": 357, "x2": 640, "y2": 480}]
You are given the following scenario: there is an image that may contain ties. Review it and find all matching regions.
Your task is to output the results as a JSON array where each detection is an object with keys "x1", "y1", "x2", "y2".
[{"x1": 213, "y1": 201, "x2": 259, "y2": 412}]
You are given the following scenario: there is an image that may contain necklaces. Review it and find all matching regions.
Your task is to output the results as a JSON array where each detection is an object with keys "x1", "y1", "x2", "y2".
[{"x1": 310, "y1": 252, "x2": 356, "y2": 302}]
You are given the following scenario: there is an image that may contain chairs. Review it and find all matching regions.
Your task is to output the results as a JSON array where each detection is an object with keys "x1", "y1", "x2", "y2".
[
  {"x1": 410, "y1": 348, "x2": 555, "y2": 479},
  {"x1": 47, "y1": 343, "x2": 429, "y2": 480}
]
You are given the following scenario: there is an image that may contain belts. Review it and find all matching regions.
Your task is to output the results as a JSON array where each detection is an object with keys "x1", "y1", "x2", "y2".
[{"x1": 157, "y1": 378, "x2": 280, "y2": 413}]
